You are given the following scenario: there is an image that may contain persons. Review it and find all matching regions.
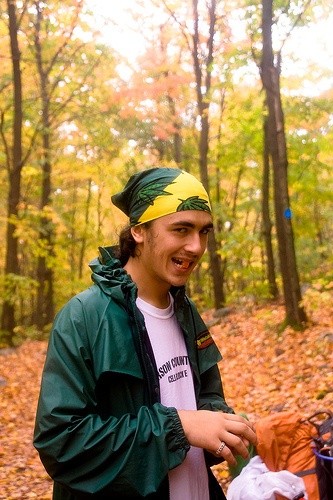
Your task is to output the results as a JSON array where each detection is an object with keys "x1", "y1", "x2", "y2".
[{"x1": 31, "y1": 167, "x2": 259, "y2": 500}]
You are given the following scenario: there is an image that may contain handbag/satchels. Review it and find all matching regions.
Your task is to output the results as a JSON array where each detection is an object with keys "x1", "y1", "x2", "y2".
[{"x1": 254, "y1": 409, "x2": 333, "y2": 500}]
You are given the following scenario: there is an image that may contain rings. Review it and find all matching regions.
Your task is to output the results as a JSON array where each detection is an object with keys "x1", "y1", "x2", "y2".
[{"x1": 215, "y1": 442, "x2": 226, "y2": 454}]
